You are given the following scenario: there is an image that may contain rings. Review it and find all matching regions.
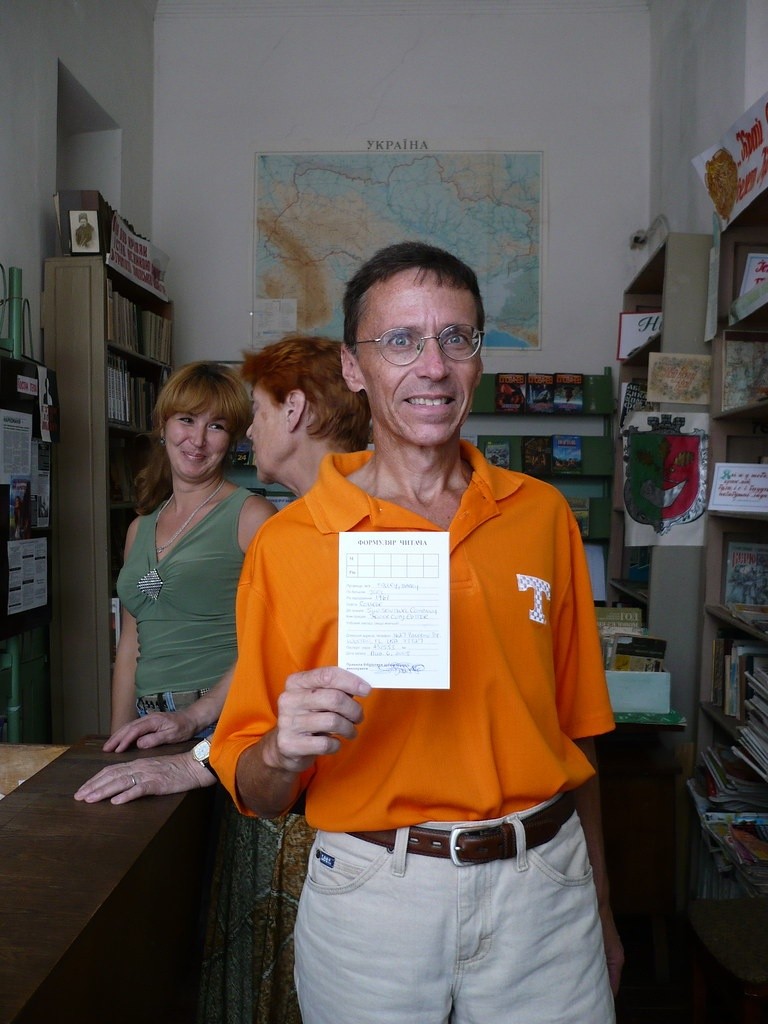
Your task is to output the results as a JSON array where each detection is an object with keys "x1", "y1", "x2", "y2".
[{"x1": 130, "y1": 775, "x2": 137, "y2": 786}]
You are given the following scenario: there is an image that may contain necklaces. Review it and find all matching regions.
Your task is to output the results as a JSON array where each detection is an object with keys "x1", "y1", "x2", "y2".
[{"x1": 153, "y1": 480, "x2": 225, "y2": 553}]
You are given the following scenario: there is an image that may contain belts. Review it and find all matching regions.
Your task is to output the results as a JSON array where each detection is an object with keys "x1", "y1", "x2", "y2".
[{"x1": 344, "y1": 793, "x2": 578, "y2": 866}]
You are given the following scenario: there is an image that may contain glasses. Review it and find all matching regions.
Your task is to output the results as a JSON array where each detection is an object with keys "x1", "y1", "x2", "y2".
[{"x1": 351, "y1": 323, "x2": 487, "y2": 367}]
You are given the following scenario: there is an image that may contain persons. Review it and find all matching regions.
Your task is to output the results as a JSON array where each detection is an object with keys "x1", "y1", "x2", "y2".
[
  {"x1": 13, "y1": 496, "x2": 29, "y2": 540},
  {"x1": 76, "y1": 334, "x2": 375, "y2": 1024},
  {"x1": 76, "y1": 213, "x2": 93, "y2": 248},
  {"x1": 211, "y1": 243, "x2": 625, "y2": 1024}
]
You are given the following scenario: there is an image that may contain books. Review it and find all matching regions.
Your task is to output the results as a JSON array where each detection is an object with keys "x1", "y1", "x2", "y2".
[
  {"x1": 722, "y1": 537, "x2": 768, "y2": 634},
  {"x1": 107, "y1": 278, "x2": 172, "y2": 363},
  {"x1": 708, "y1": 638, "x2": 768, "y2": 778},
  {"x1": 593, "y1": 606, "x2": 666, "y2": 672},
  {"x1": 686, "y1": 744, "x2": 768, "y2": 902},
  {"x1": 478, "y1": 433, "x2": 582, "y2": 475},
  {"x1": 108, "y1": 354, "x2": 164, "y2": 432},
  {"x1": 495, "y1": 374, "x2": 583, "y2": 416}
]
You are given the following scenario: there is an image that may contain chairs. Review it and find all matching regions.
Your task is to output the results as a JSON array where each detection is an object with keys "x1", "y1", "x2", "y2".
[{"x1": 683, "y1": 899, "x2": 768, "y2": 1024}]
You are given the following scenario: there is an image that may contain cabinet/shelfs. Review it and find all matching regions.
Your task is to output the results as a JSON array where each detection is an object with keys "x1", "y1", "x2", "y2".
[
  {"x1": 41, "y1": 255, "x2": 172, "y2": 742},
  {"x1": 603, "y1": 234, "x2": 711, "y2": 723},
  {"x1": 690, "y1": 169, "x2": 768, "y2": 898},
  {"x1": 221, "y1": 366, "x2": 617, "y2": 540}
]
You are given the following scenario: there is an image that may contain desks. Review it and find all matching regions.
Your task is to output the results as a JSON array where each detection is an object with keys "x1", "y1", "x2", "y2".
[
  {"x1": 593, "y1": 709, "x2": 687, "y2": 979},
  {"x1": 0, "y1": 736, "x2": 221, "y2": 1024}
]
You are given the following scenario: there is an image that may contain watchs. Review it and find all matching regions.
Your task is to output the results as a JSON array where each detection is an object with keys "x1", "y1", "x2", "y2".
[{"x1": 190, "y1": 737, "x2": 223, "y2": 783}]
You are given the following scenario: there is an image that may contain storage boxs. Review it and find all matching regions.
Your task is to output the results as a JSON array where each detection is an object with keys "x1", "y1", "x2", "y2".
[{"x1": 605, "y1": 665, "x2": 671, "y2": 715}]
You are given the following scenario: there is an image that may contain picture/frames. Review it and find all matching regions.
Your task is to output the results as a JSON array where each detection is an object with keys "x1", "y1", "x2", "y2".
[{"x1": 68, "y1": 208, "x2": 102, "y2": 255}]
